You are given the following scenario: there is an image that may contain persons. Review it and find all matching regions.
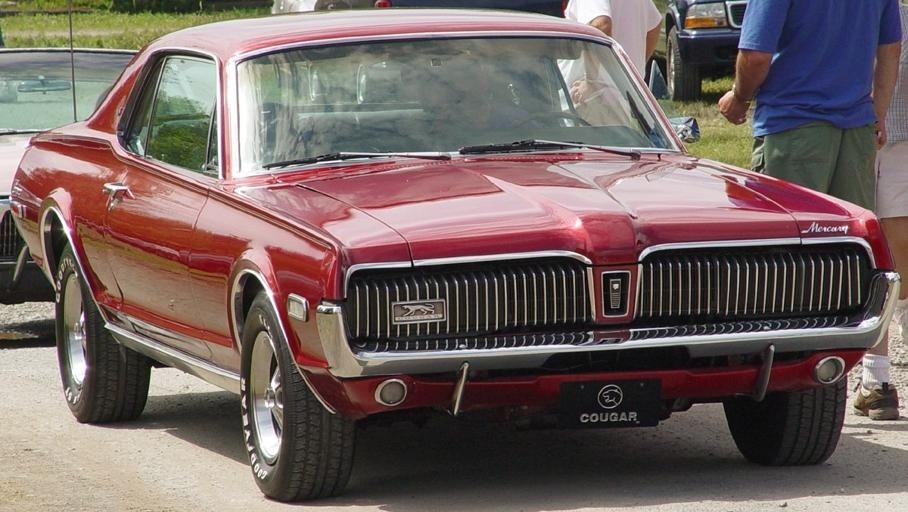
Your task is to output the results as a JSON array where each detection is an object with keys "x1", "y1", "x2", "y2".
[
  {"x1": 415, "y1": 58, "x2": 549, "y2": 152},
  {"x1": 557, "y1": 1, "x2": 666, "y2": 126},
  {"x1": 851, "y1": 1, "x2": 908, "y2": 422},
  {"x1": 714, "y1": 1, "x2": 903, "y2": 216}
]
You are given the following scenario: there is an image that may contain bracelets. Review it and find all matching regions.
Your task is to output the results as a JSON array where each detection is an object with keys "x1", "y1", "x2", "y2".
[
  {"x1": 731, "y1": 84, "x2": 751, "y2": 105},
  {"x1": 584, "y1": 75, "x2": 600, "y2": 84}
]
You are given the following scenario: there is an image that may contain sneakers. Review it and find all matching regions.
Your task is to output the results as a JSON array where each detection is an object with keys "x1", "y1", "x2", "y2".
[{"x1": 852, "y1": 384, "x2": 901, "y2": 423}]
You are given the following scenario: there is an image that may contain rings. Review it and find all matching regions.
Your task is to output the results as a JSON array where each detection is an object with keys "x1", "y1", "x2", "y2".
[{"x1": 576, "y1": 92, "x2": 579, "y2": 95}]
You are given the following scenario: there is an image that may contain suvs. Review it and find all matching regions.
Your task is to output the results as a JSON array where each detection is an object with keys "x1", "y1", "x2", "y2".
[{"x1": 649, "y1": 1, "x2": 754, "y2": 105}]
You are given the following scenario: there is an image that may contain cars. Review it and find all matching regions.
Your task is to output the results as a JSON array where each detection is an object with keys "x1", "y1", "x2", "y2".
[
  {"x1": 6, "y1": 6, "x2": 904, "y2": 505},
  {"x1": 1, "y1": 44, "x2": 142, "y2": 311},
  {"x1": 270, "y1": 0, "x2": 421, "y2": 110}
]
